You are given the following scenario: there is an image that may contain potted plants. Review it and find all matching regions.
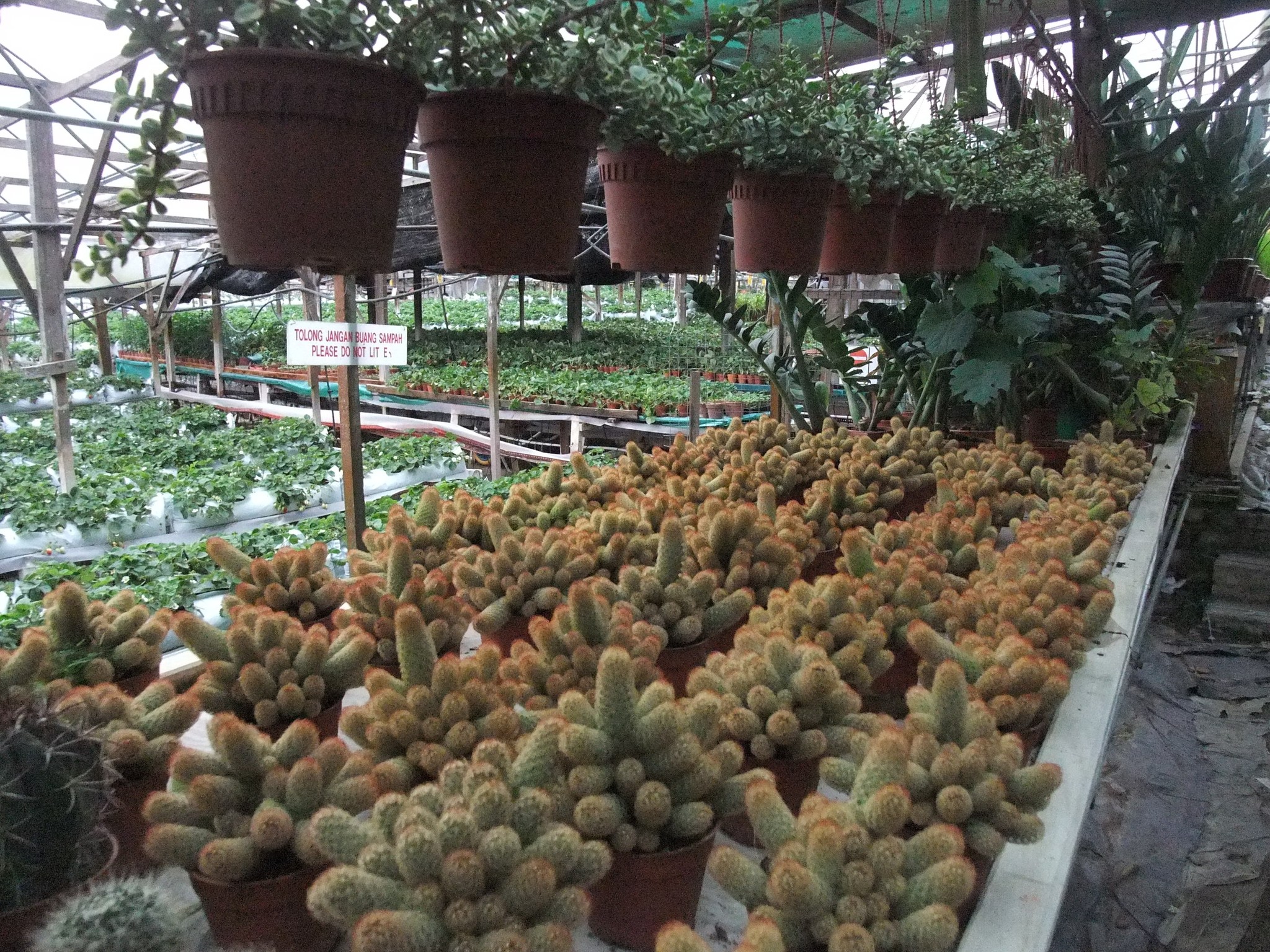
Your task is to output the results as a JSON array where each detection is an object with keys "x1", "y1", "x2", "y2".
[{"x1": 1, "y1": 1, "x2": 1269, "y2": 951}]
